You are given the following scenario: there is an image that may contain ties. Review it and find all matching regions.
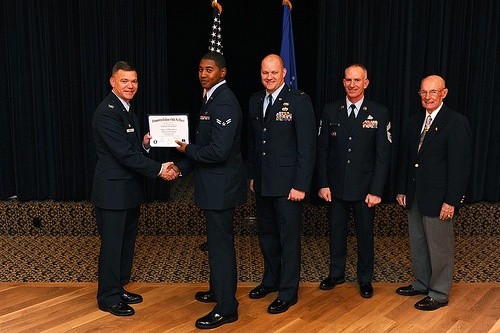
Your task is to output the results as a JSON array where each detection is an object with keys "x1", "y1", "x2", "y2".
[
  {"x1": 202, "y1": 94, "x2": 207, "y2": 105},
  {"x1": 417, "y1": 115, "x2": 432, "y2": 153},
  {"x1": 265, "y1": 94, "x2": 272, "y2": 119},
  {"x1": 348, "y1": 105, "x2": 356, "y2": 131},
  {"x1": 128, "y1": 106, "x2": 134, "y2": 127}
]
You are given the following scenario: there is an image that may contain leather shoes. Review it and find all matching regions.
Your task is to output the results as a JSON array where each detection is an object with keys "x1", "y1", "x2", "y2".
[
  {"x1": 98, "y1": 301, "x2": 135, "y2": 316},
  {"x1": 195, "y1": 291, "x2": 217, "y2": 302},
  {"x1": 268, "y1": 297, "x2": 298, "y2": 314},
  {"x1": 319, "y1": 277, "x2": 345, "y2": 290},
  {"x1": 120, "y1": 287, "x2": 143, "y2": 304},
  {"x1": 415, "y1": 296, "x2": 448, "y2": 311},
  {"x1": 396, "y1": 285, "x2": 429, "y2": 296},
  {"x1": 359, "y1": 282, "x2": 373, "y2": 298},
  {"x1": 195, "y1": 309, "x2": 238, "y2": 329},
  {"x1": 249, "y1": 284, "x2": 279, "y2": 299}
]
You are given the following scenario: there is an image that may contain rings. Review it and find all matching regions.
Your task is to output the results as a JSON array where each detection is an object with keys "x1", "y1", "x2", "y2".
[{"x1": 448, "y1": 215, "x2": 450, "y2": 217}]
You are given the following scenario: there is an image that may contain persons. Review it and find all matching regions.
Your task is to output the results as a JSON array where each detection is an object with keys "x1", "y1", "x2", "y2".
[
  {"x1": 280, "y1": 0, "x2": 297, "y2": 89},
  {"x1": 91, "y1": 61, "x2": 179, "y2": 316},
  {"x1": 317, "y1": 64, "x2": 393, "y2": 297},
  {"x1": 248, "y1": 54, "x2": 317, "y2": 312},
  {"x1": 393, "y1": 75, "x2": 473, "y2": 310},
  {"x1": 167, "y1": 52, "x2": 248, "y2": 328}
]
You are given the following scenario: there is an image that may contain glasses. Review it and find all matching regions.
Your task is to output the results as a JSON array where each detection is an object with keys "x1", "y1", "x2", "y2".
[{"x1": 418, "y1": 88, "x2": 446, "y2": 97}]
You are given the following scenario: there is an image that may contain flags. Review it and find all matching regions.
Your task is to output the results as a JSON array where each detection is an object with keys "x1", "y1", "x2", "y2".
[{"x1": 208, "y1": 0, "x2": 224, "y2": 55}]
